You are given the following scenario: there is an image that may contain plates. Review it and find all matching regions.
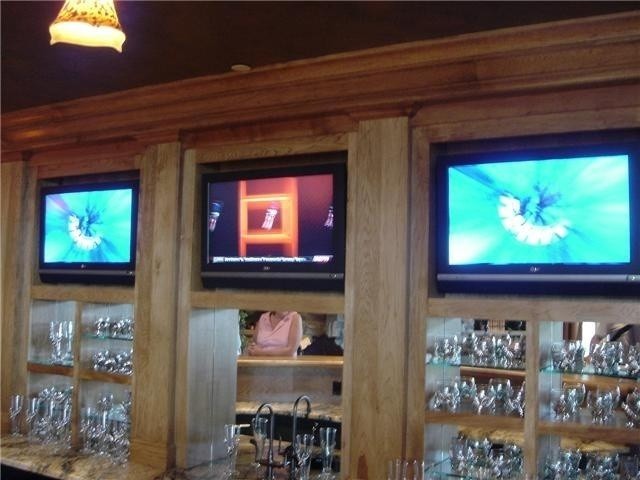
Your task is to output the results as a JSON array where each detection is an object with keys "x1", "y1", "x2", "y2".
[
  {"x1": 423, "y1": 297, "x2": 640, "y2": 471},
  {"x1": 27, "y1": 287, "x2": 135, "y2": 447}
]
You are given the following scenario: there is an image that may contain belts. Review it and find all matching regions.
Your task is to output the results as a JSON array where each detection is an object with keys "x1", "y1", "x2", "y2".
[
  {"x1": 5, "y1": 394, "x2": 128, "y2": 465},
  {"x1": 224, "y1": 418, "x2": 338, "y2": 480},
  {"x1": 49, "y1": 321, "x2": 75, "y2": 361}
]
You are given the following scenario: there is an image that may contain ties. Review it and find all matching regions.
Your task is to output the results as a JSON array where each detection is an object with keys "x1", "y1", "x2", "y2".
[
  {"x1": 435, "y1": 139, "x2": 640, "y2": 298},
  {"x1": 200, "y1": 162, "x2": 348, "y2": 293},
  {"x1": 39, "y1": 179, "x2": 141, "y2": 285}
]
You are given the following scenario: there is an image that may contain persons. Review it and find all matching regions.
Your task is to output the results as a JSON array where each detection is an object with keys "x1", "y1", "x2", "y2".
[
  {"x1": 585, "y1": 323, "x2": 640, "y2": 363},
  {"x1": 247, "y1": 310, "x2": 344, "y2": 356}
]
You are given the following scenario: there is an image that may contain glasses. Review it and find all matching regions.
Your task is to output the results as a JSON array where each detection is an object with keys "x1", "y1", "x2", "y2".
[{"x1": 49, "y1": 0, "x2": 127, "y2": 53}]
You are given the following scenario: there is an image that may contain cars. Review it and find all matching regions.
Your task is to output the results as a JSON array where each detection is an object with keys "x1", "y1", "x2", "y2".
[{"x1": 430, "y1": 322, "x2": 635, "y2": 477}]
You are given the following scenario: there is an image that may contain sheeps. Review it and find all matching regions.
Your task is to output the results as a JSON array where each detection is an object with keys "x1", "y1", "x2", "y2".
[
  {"x1": 292, "y1": 396, "x2": 311, "y2": 447},
  {"x1": 255, "y1": 403, "x2": 274, "y2": 450}
]
